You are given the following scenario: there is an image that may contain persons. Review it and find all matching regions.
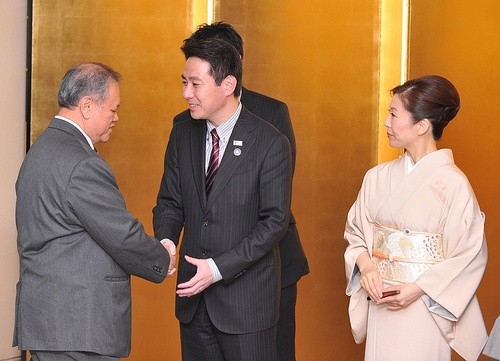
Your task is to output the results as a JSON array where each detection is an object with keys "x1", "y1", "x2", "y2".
[
  {"x1": 152, "y1": 20, "x2": 310, "y2": 361},
  {"x1": 344, "y1": 75, "x2": 489, "y2": 361},
  {"x1": 12, "y1": 62, "x2": 177, "y2": 361},
  {"x1": 483, "y1": 316, "x2": 500, "y2": 361}
]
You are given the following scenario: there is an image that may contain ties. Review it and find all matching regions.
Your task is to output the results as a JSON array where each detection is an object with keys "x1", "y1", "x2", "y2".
[{"x1": 205, "y1": 128, "x2": 220, "y2": 202}]
